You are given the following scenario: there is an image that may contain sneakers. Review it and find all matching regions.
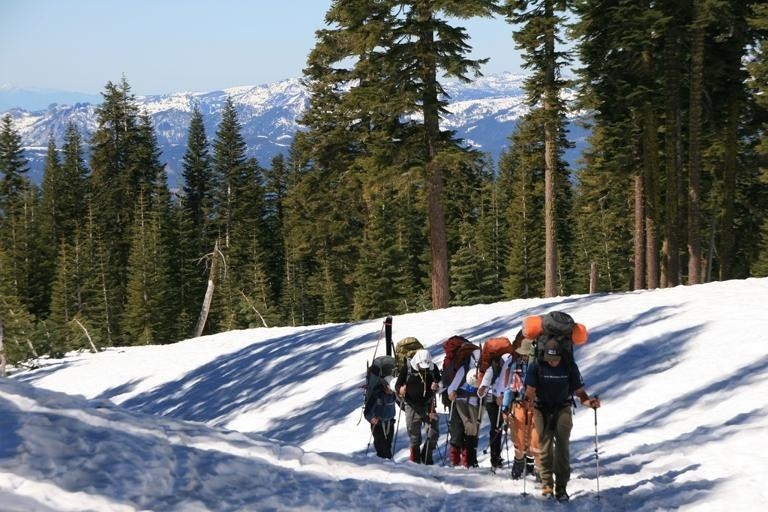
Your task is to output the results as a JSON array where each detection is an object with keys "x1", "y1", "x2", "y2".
[
  {"x1": 490, "y1": 456, "x2": 505, "y2": 467},
  {"x1": 512, "y1": 456, "x2": 568, "y2": 503}
]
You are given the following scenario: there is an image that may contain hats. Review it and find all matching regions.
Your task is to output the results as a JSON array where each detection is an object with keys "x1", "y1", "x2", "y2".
[
  {"x1": 382, "y1": 375, "x2": 394, "y2": 386},
  {"x1": 418, "y1": 360, "x2": 431, "y2": 368}
]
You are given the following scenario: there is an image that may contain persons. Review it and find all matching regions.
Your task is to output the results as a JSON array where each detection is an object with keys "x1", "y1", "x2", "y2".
[
  {"x1": 395, "y1": 348, "x2": 441, "y2": 465},
  {"x1": 363, "y1": 376, "x2": 405, "y2": 460},
  {"x1": 448, "y1": 338, "x2": 600, "y2": 503}
]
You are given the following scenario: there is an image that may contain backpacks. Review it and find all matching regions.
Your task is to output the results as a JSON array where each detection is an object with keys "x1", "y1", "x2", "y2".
[
  {"x1": 394, "y1": 337, "x2": 435, "y2": 402},
  {"x1": 475, "y1": 311, "x2": 588, "y2": 410},
  {"x1": 363, "y1": 356, "x2": 396, "y2": 405},
  {"x1": 441, "y1": 335, "x2": 480, "y2": 407}
]
